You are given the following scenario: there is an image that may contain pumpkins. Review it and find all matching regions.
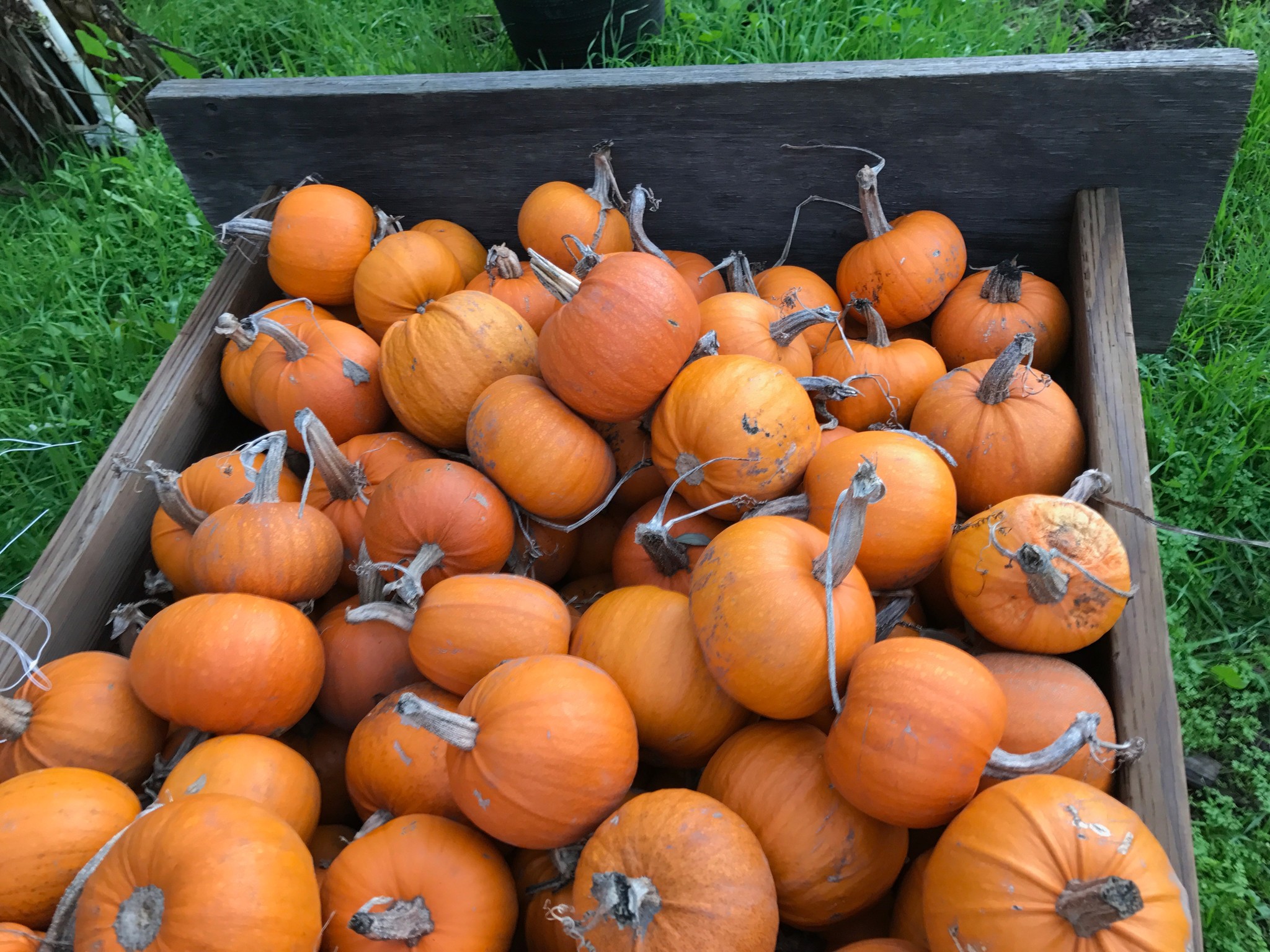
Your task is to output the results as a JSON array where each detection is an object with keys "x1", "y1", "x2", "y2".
[{"x1": 2, "y1": 141, "x2": 1194, "y2": 950}]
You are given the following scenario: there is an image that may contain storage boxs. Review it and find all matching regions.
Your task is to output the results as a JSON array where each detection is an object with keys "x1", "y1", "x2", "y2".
[{"x1": 2, "y1": 47, "x2": 1260, "y2": 952}]
